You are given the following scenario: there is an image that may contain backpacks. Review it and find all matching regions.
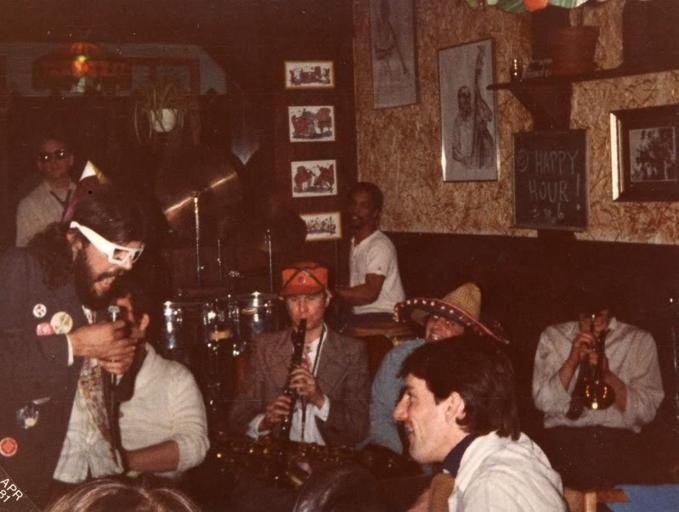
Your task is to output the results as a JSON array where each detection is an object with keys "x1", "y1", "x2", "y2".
[
  {"x1": 235, "y1": 292, "x2": 280, "y2": 340},
  {"x1": 202, "y1": 294, "x2": 246, "y2": 358},
  {"x1": 150, "y1": 292, "x2": 206, "y2": 352}
]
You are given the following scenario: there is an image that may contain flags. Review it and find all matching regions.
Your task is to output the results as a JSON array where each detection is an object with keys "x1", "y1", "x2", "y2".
[{"x1": 546, "y1": 27, "x2": 599, "y2": 78}]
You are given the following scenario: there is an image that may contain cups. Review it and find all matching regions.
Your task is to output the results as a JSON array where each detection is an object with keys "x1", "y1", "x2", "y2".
[
  {"x1": 68, "y1": 220, "x2": 143, "y2": 265},
  {"x1": 39, "y1": 150, "x2": 67, "y2": 162}
]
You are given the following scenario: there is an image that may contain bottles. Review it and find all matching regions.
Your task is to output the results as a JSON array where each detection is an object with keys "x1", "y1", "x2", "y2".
[
  {"x1": 280, "y1": 260, "x2": 328, "y2": 297},
  {"x1": 393, "y1": 282, "x2": 499, "y2": 341}
]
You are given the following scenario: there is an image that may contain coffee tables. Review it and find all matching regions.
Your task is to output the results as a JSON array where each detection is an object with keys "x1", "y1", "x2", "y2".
[
  {"x1": 155, "y1": 154, "x2": 242, "y2": 239},
  {"x1": 225, "y1": 210, "x2": 307, "y2": 270}
]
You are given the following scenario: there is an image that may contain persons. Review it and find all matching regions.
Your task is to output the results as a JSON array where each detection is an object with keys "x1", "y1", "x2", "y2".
[
  {"x1": 531, "y1": 267, "x2": 664, "y2": 491},
  {"x1": 288, "y1": 213, "x2": 311, "y2": 267},
  {"x1": 393, "y1": 337, "x2": 568, "y2": 511},
  {"x1": 339, "y1": 183, "x2": 406, "y2": 316},
  {"x1": 229, "y1": 265, "x2": 368, "y2": 442},
  {"x1": 16, "y1": 129, "x2": 85, "y2": 246},
  {"x1": 452, "y1": 86, "x2": 492, "y2": 161},
  {"x1": 630, "y1": 128, "x2": 676, "y2": 182},
  {"x1": 102, "y1": 263, "x2": 209, "y2": 478},
  {"x1": 369, "y1": 281, "x2": 510, "y2": 472},
  {"x1": 0, "y1": 185, "x2": 163, "y2": 486},
  {"x1": 48, "y1": 474, "x2": 203, "y2": 512}
]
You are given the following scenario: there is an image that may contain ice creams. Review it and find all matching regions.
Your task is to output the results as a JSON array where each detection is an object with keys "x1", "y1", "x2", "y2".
[{"x1": 563, "y1": 312, "x2": 617, "y2": 424}]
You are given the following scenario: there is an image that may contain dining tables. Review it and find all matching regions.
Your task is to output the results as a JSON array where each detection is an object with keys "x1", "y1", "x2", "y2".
[{"x1": 134, "y1": 72, "x2": 197, "y2": 131}]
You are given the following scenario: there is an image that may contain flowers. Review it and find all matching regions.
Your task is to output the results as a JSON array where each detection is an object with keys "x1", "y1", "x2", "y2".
[{"x1": 104, "y1": 304, "x2": 122, "y2": 389}]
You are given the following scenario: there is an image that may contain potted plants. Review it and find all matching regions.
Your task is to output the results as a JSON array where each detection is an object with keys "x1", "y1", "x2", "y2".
[
  {"x1": 367, "y1": 1, "x2": 420, "y2": 110},
  {"x1": 288, "y1": 105, "x2": 336, "y2": 144},
  {"x1": 291, "y1": 159, "x2": 338, "y2": 198},
  {"x1": 297, "y1": 211, "x2": 343, "y2": 242},
  {"x1": 436, "y1": 38, "x2": 500, "y2": 182},
  {"x1": 284, "y1": 61, "x2": 336, "y2": 90},
  {"x1": 609, "y1": 104, "x2": 679, "y2": 202}
]
